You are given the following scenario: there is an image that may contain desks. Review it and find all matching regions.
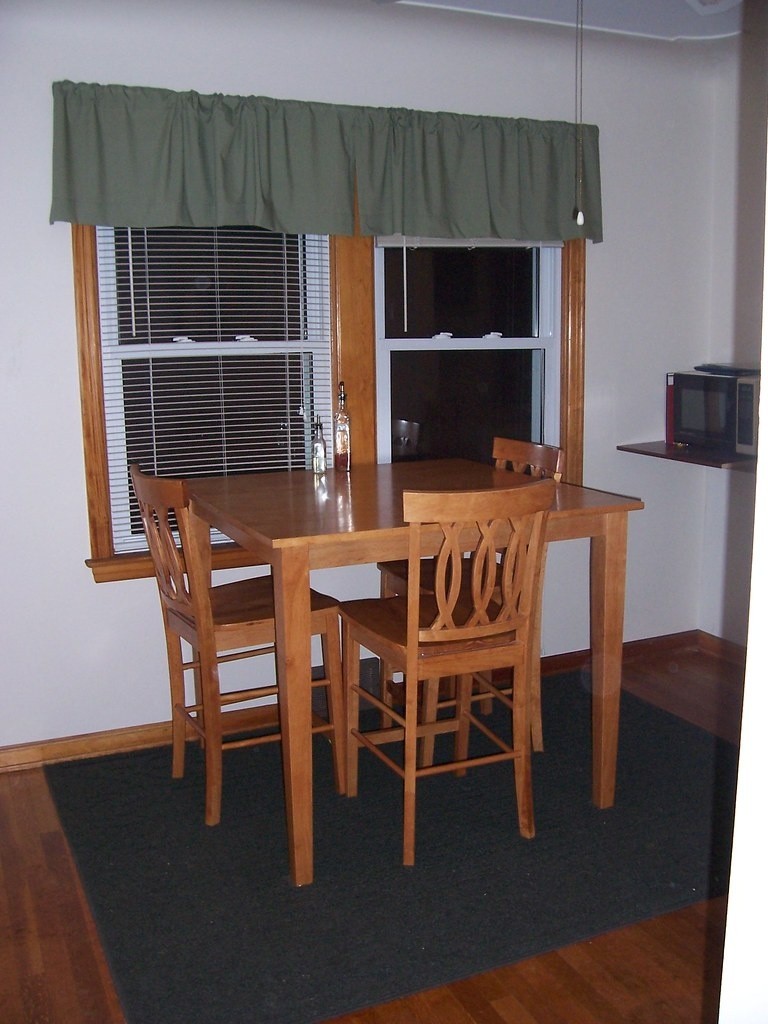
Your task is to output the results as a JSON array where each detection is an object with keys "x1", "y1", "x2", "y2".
[{"x1": 173, "y1": 457, "x2": 645, "y2": 887}]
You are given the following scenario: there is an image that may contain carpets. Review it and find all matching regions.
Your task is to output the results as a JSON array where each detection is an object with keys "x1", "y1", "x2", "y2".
[{"x1": 43, "y1": 666, "x2": 739, "y2": 1024}]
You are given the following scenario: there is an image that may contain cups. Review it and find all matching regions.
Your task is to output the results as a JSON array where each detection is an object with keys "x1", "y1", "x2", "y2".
[{"x1": 391, "y1": 418, "x2": 420, "y2": 457}]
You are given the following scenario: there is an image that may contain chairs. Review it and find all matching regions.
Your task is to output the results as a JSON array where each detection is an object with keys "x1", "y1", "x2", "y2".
[
  {"x1": 374, "y1": 437, "x2": 564, "y2": 771},
  {"x1": 336, "y1": 477, "x2": 556, "y2": 867},
  {"x1": 128, "y1": 464, "x2": 355, "y2": 827}
]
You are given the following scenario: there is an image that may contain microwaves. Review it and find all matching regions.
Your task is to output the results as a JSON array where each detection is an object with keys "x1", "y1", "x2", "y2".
[{"x1": 665, "y1": 371, "x2": 760, "y2": 457}]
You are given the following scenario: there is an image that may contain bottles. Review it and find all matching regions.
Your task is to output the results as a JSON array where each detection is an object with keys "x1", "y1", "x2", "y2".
[
  {"x1": 334, "y1": 381, "x2": 351, "y2": 472},
  {"x1": 311, "y1": 414, "x2": 327, "y2": 474}
]
